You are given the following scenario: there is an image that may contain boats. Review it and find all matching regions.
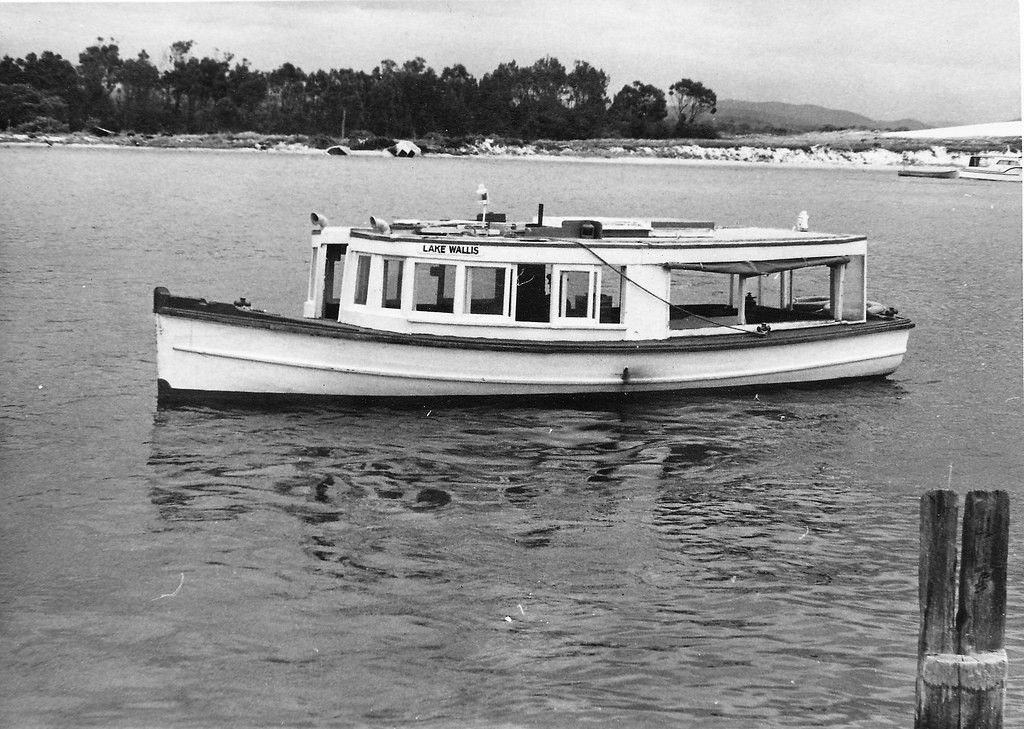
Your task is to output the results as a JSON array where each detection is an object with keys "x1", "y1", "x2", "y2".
[
  {"x1": 897, "y1": 169, "x2": 958, "y2": 179},
  {"x1": 153, "y1": 182, "x2": 917, "y2": 407},
  {"x1": 957, "y1": 157, "x2": 1023, "y2": 182}
]
